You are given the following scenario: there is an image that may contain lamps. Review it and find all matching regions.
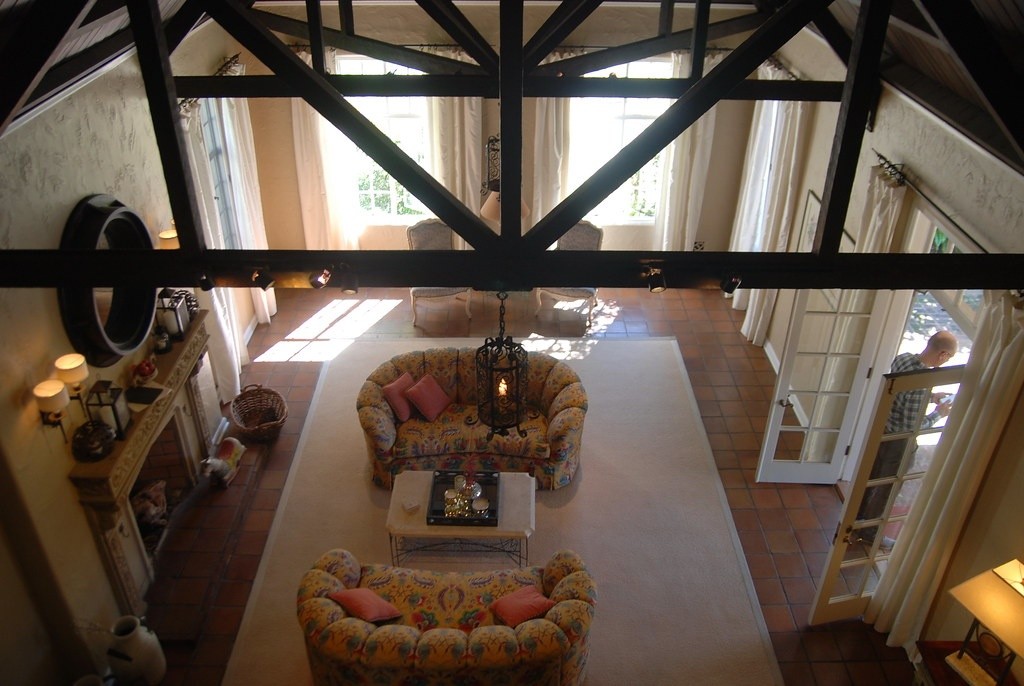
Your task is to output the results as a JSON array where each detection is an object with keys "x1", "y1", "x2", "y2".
[
  {"x1": 33, "y1": 354, "x2": 89, "y2": 443},
  {"x1": 718, "y1": 275, "x2": 741, "y2": 294},
  {"x1": 480, "y1": 133, "x2": 531, "y2": 221},
  {"x1": 195, "y1": 267, "x2": 216, "y2": 292},
  {"x1": 647, "y1": 266, "x2": 666, "y2": 294},
  {"x1": 463, "y1": 293, "x2": 541, "y2": 441},
  {"x1": 341, "y1": 273, "x2": 359, "y2": 297},
  {"x1": 944, "y1": 558, "x2": 1024, "y2": 686},
  {"x1": 159, "y1": 218, "x2": 180, "y2": 249},
  {"x1": 309, "y1": 267, "x2": 332, "y2": 291},
  {"x1": 249, "y1": 270, "x2": 275, "y2": 292}
]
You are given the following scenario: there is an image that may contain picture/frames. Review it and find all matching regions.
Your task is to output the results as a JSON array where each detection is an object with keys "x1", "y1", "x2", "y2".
[{"x1": 796, "y1": 189, "x2": 856, "y2": 311}]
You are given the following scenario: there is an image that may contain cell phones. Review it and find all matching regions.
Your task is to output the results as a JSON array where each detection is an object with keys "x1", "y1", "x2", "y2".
[{"x1": 939, "y1": 394, "x2": 956, "y2": 405}]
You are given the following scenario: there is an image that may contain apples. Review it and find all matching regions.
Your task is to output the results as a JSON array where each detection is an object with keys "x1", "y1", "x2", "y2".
[{"x1": 137, "y1": 360, "x2": 155, "y2": 376}]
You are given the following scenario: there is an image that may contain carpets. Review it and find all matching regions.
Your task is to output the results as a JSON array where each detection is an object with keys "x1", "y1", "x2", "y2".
[{"x1": 225, "y1": 337, "x2": 786, "y2": 686}]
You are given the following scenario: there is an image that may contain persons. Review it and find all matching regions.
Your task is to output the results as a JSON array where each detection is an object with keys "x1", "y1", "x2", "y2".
[{"x1": 853, "y1": 331, "x2": 957, "y2": 549}]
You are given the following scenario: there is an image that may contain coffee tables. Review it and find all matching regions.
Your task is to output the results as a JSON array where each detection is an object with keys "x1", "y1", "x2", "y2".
[{"x1": 385, "y1": 471, "x2": 537, "y2": 567}]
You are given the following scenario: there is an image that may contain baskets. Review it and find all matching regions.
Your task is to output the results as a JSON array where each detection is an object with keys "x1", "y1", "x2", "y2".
[{"x1": 230, "y1": 383, "x2": 287, "y2": 439}]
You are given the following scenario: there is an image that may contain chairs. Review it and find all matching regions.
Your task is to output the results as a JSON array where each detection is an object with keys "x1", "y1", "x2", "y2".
[
  {"x1": 406, "y1": 218, "x2": 473, "y2": 326},
  {"x1": 535, "y1": 220, "x2": 603, "y2": 328}
]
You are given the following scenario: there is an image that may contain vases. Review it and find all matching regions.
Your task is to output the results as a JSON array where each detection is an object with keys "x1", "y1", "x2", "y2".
[{"x1": 107, "y1": 615, "x2": 167, "y2": 686}]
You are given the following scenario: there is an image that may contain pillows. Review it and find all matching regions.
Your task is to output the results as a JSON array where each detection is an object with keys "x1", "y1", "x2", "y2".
[
  {"x1": 327, "y1": 587, "x2": 403, "y2": 623},
  {"x1": 489, "y1": 586, "x2": 557, "y2": 627},
  {"x1": 404, "y1": 373, "x2": 452, "y2": 421},
  {"x1": 382, "y1": 373, "x2": 416, "y2": 422}
]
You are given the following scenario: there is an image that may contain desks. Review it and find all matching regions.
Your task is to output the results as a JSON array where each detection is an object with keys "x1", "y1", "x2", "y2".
[{"x1": 911, "y1": 640, "x2": 1021, "y2": 686}]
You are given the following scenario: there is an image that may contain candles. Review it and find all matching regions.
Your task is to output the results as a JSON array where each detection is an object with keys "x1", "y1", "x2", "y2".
[
  {"x1": 86, "y1": 381, "x2": 134, "y2": 440},
  {"x1": 155, "y1": 288, "x2": 191, "y2": 341}
]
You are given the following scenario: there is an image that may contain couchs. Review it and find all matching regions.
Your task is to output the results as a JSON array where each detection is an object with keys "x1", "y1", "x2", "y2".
[
  {"x1": 297, "y1": 548, "x2": 597, "y2": 686},
  {"x1": 358, "y1": 347, "x2": 589, "y2": 490}
]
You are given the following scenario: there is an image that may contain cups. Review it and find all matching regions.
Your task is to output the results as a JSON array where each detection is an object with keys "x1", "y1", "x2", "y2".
[
  {"x1": 444, "y1": 489, "x2": 458, "y2": 506},
  {"x1": 454, "y1": 475, "x2": 466, "y2": 493},
  {"x1": 471, "y1": 498, "x2": 489, "y2": 519}
]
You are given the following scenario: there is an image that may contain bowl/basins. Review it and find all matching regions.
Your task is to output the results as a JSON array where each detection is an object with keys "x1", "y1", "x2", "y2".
[{"x1": 138, "y1": 368, "x2": 158, "y2": 383}]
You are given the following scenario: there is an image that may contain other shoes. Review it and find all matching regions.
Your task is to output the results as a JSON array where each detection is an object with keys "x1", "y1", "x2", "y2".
[{"x1": 862, "y1": 536, "x2": 894, "y2": 549}]
